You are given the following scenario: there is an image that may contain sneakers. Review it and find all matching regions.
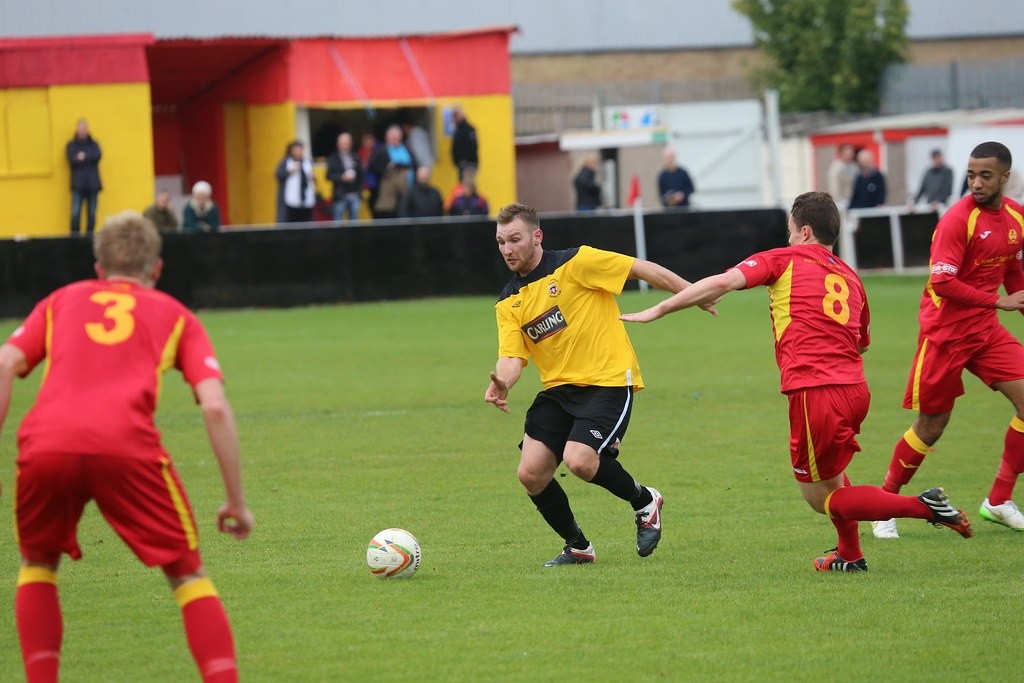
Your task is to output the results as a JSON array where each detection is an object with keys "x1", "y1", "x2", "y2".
[
  {"x1": 871, "y1": 518, "x2": 900, "y2": 539},
  {"x1": 917, "y1": 487, "x2": 972, "y2": 539},
  {"x1": 541, "y1": 541, "x2": 596, "y2": 567},
  {"x1": 978, "y1": 498, "x2": 1024, "y2": 531},
  {"x1": 633, "y1": 485, "x2": 663, "y2": 557},
  {"x1": 812, "y1": 547, "x2": 867, "y2": 571}
]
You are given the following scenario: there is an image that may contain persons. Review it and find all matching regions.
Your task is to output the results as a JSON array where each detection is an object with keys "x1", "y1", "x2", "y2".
[
  {"x1": 827, "y1": 143, "x2": 887, "y2": 209},
  {"x1": 66, "y1": 119, "x2": 102, "y2": 235},
  {"x1": 182, "y1": 182, "x2": 220, "y2": 231},
  {"x1": 276, "y1": 141, "x2": 316, "y2": 222},
  {"x1": 142, "y1": 191, "x2": 177, "y2": 232},
  {"x1": 574, "y1": 156, "x2": 601, "y2": 211},
  {"x1": 617, "y1": 191, "x2": 973, "y2": 572},
  {"x1": 0, "y1": 214, "x2": 256, "y2": 683},
  {"x1": 871, "y1": 142, "x2": 1024, "y2": 538},
  {"x1": 913, "y1": 150, "x2": 969, "y2": 209},
  {"x1": 326, "y1": 121, "x2": 444, "y2": 222},
  {"x1": 485, "y1": 204, "x2": 723, "y2": 567},
  {"x1": 658, "y1": 148, "x2": 694, "y2": 210},
  {"x1": 447, "y1": 108, "x2": 487, "y2": 215}
]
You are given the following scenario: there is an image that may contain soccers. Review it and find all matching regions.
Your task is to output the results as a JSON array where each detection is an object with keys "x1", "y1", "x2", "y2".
[{"x1": 367, "y1": 527, "x2": 422, "y2": 578}]
